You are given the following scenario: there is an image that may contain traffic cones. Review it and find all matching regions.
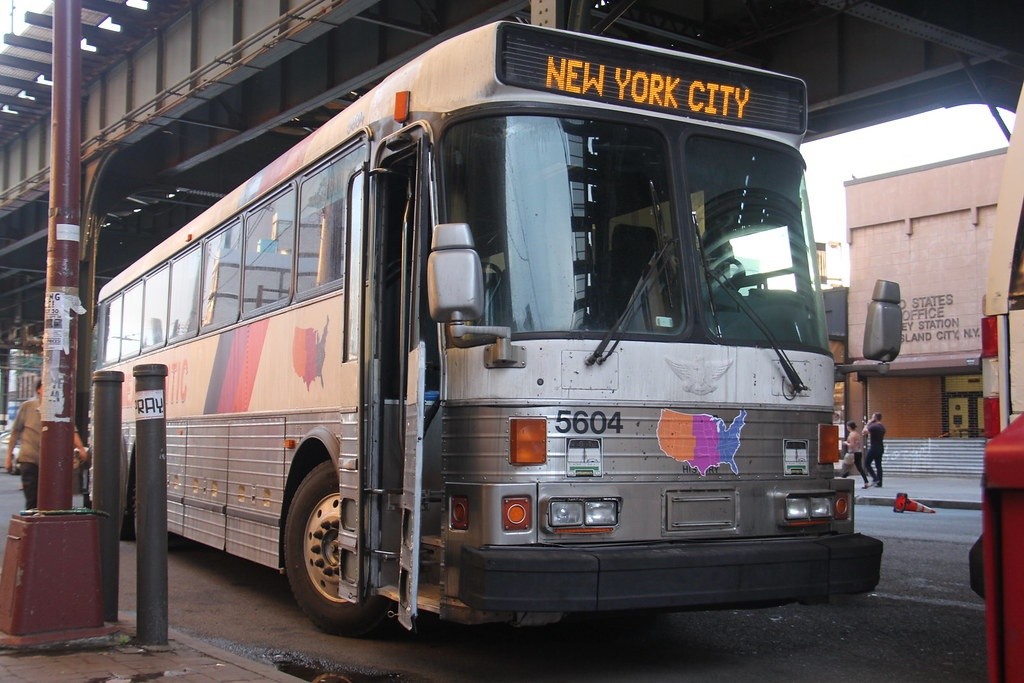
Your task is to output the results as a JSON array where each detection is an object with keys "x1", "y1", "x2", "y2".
[{"x1": 894, "y1": 493, "x2": 936, "y2": 514}]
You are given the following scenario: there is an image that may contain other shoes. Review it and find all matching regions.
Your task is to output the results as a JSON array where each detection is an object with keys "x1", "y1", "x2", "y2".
[
  {"x1": 871, "y1": 480, "x2": 880, "y2": 486},
  {"x1": 862, "y1": 484, "x2": 870, "y2": 489},
  {"x1": 875, "y1": 483, "x2": 881, "y2": 487}
]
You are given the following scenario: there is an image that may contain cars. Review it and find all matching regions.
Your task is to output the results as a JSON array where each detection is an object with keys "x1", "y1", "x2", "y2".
[{"x1": 2, "y1": 430, "x2": 23, "y2": 476}]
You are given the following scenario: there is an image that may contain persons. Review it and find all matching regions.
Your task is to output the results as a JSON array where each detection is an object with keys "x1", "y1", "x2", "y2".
[
  {"x1": 863, "y1": 412, "x2": 886, "y2": 488},
  {"x1": 840, "y1": 420, "x2": 870, "y2": 488},
  {"x1": 5, "y1": 377, "x2": 88, "y2": 510}
]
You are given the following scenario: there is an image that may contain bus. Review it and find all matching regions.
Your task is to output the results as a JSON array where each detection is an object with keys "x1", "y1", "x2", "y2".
[
  {"x1": 968, "y1": 80, "x2": 1024, "y2": 600},
  {"x1": 89, "y1": 20, "x2": 902, "y2": 639}
]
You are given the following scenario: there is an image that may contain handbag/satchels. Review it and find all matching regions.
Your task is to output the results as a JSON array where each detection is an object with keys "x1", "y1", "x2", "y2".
[{"x1": 844, "y1": 453, "x2": 855, "y2": 464}]
